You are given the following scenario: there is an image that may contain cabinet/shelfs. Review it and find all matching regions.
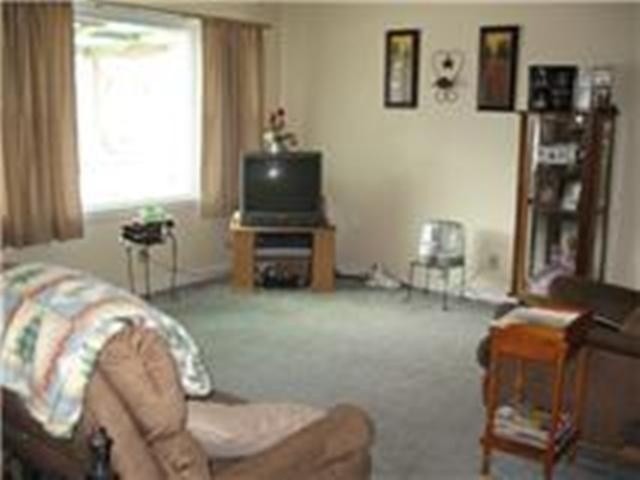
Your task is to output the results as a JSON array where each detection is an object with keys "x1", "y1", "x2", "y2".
[
  {"x1": 228, "y1": 211, "x2": 336, "y2": 291},
  {"x1": 510, "y1": 103, "x2": 620, "y2": 306}
]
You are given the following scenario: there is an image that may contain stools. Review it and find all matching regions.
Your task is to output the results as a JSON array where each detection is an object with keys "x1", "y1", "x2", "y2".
[{"x1": 117, "y1": 223, "x2": 178, "y2": 302}]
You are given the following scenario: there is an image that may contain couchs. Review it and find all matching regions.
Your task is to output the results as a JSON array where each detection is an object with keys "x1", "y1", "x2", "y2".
[{"x1": 0, "y1": 262, "x2": 376, "y2": 480}]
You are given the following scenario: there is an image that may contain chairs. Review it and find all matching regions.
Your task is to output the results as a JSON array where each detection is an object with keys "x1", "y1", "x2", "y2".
[
  {"x1": 476, "y1": 274, "x2": 640, "y2": 464},
  {"x1": 403, "y1": 220, "x2": 465, "y2": 311}
]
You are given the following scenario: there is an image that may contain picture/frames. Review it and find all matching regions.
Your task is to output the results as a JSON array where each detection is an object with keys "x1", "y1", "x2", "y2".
[
  {"x1": 477, "y1": 27, "x2": 519, "y2": 111},
  {"x1": 384, "y1": 29, "x2": 418, "y2": 109}
]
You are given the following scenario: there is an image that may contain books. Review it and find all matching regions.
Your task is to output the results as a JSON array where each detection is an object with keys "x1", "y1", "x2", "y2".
[{"x1": 493, "y1": 396, "x2": 576, "y2": 451}]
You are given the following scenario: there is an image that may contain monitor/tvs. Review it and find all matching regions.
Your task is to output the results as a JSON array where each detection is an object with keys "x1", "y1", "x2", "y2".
[{"x1": 239, "y1": 149, "x2": 322, "y2": 229}]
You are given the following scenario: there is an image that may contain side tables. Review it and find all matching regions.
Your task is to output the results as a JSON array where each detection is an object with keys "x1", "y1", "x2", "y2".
[{"x1": 480, "y1": 305, "x2": 595, "y2": 480}]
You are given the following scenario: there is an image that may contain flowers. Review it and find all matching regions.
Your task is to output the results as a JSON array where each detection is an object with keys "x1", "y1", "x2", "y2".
[{"x1": 263, "y1": 108, "x2": 299, "y2": 146}]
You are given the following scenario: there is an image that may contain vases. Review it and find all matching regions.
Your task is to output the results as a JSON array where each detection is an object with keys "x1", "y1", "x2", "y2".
[{"x1": 270, "y1": 142, "x2": 287, "y2": 154}]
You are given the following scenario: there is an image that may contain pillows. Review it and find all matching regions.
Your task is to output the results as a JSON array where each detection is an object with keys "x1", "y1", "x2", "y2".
[{"x1": 185, "y1": 397, "x2": 329, "y2": 459}]
